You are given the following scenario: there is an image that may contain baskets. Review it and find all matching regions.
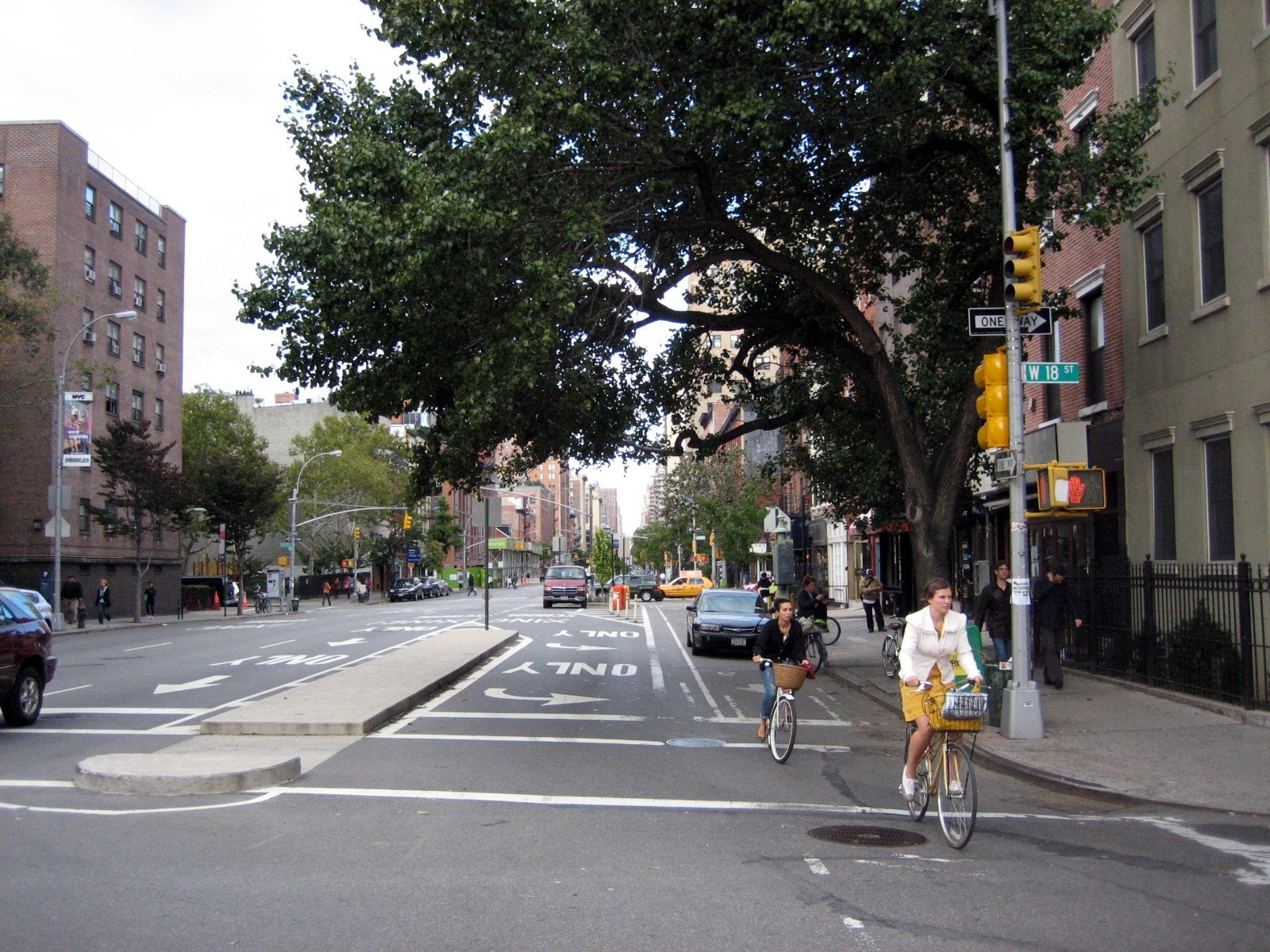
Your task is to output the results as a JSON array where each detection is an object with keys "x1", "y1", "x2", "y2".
[
  {"x1": 924, "y1": 693, "x2": 986, "y2": 733},
  {"x1": 772, "y1": 663, "x2": 808, "y2": 691}
]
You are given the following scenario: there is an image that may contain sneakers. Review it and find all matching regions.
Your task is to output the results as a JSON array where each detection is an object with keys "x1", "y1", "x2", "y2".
[
  {"x1": 901, "y1": 764, "x2": 919, "y2": 802},
  {"x1": 948, "y1": 780, "x2": 965, "y2": 796}
]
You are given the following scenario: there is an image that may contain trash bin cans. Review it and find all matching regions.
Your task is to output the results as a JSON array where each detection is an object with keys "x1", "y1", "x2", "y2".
[
  {"x1": 291, "y1": 598, "x2": 300, "y2": 611},
  {"x1": 358, "y1": 592, "x2": 365, "y2": 603},
  {"x1": 948, "y1": 625, "x2": 988, "y2": 700}
]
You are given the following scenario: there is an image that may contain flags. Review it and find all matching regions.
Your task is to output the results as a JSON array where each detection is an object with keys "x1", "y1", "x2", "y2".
[
  {"x1": 62, "y1": 392, "x2": 95, "y2": 469},
  {"x1": 218, "y1": 524, "x2": 225, "y2": 565}
]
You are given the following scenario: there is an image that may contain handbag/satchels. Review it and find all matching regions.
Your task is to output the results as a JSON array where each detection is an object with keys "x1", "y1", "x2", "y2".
[
  {"x1": 141, "y1": 594, "x2": 148, "y2": 600},
  {"x1": 860, "y1": 593, "x2": 863, "y2": 601},
  {"x1": 344, "y1": 584, "x2": 349, "y2": 589}
]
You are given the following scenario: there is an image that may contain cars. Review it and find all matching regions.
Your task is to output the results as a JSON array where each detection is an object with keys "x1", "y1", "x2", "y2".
[
  {"x1": 654, "y1": 577, "x2": 714, "y2": 602},
  {"x1": 686, "y1": 588, "x2": 774, "y2": 656},
  {"x1": 0, "y1": 586, "x2": 58, "y2": 728},
  {"x1": 389, "y1": 576, "x2": 449, "y2": 602}
]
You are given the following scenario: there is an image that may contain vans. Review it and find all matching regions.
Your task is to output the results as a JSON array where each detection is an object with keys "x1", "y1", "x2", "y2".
[{"x1": 181, "y1": 576, "x2": 240, "y2": 605}]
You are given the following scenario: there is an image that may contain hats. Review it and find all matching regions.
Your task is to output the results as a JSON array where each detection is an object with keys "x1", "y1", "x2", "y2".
[
  {"x1": 865, "y1": 568, "x2": 874, "y2": 576},
  {"x1": 67, "y1": 575, "x2": 75, "y2": 579}
]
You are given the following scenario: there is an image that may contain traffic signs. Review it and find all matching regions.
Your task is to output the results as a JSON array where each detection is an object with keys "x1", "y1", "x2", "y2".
[
  {"x1": 994, "y1": 448, "x2": 1019, "y2": 480},
  {"x1": 1021, "y1": 362, "x2": 1080, "y2": 384},
  {"x1": 968, "y1": 307, "x2": 1052, "y2": 336}
]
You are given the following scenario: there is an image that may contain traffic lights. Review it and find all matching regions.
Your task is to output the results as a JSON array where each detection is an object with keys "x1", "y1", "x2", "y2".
[
  {"x1": 1004, "y1": 225, "x2": 1043, "y2": 316},
  {"x1": 1035, "y1": 466, "x2": 1106, "y2": 510},
  {"x1": 586, "y1": 560, "x2": 590, "y2": 564},
  {"x1": 403, "y1": 514, "x2": 412, "y2": 530},
  {"x1": 515, "y1": 541, "x2": 526, "y2": 549},
  {"x1": 574, "y1": 554, "x2": 578, "y2": 558},
  {"x1": 974, "y1": 345, "x2": 1009, "y2": 451},
  {"x1": 354, "y1": 527, "x2": 361, "y2": 540}
]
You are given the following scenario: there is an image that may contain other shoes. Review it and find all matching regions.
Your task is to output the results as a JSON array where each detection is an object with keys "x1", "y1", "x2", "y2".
[
  {"x1": 878, "y1": 627, "x2": 887, "y2": 632},
  {"x1": 108, "y1": 618, "x2": 113, "y2": 623},
  {"x1": 787, "y1": 715, "x2": 791, "y2": 723},
  {"x1": 67, "y1": 620, "x2": 77, "y2": 624},
  {"x1": 869, "y1": 629, "x2": 873, "y2": 632},
  {"x1": 99, "y1": 622, "x2": 103, "y2": 625},
  {"x1": 1053, "y1": 678, "x2": 1064, "y2": 688},
  {"x1": 823, "y1": 659, "x2": 833, "y2": 667},
  {"x1": 1044, "y1": 681, "x2": 1054, "y2": 686},
  {"x1": 756, "y1": 725, "x2": 767, "y2": 743},
  {"x1": 147, "y1": 614, "x2": 154, "y2": 619}
]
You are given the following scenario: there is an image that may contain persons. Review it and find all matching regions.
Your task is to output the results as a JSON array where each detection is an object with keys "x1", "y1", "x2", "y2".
[
  {"x1": 858, "y1": 568, "x2": 889, "y2": 633},
  {"x1": 252, "y1": 585, "x2": 265, "y2": 612},
  {"x1": 1030, "y1": 566, "x2": 1084, "y2": 689},
  {"x1": 897, "y1": 578, "x2": 984, "y2": 802},
  {"x1": 60, "y1": 575, "x2": 84, "y2": 624},
  {"x1": 973, "y1": 562, "x2": 1012, "y2": 671},
  {"x1": 144, "y1": 582, "x2": 157, "y2": 618},
  {"x1": 94, "y1": 578, "x2": 113, "y2": 624},
  {"x1": 319, "y1": 559, "x2": 553, "y2": 608},
  {"x1": 796, "y1": 576, "x2": 834, "y2": 669},
  {"x1": 753, "y1": 596, "x2": 810, "y2": 744},
  {"x1": 756, "y1": 572, "x2": 772, "y2": 598}
]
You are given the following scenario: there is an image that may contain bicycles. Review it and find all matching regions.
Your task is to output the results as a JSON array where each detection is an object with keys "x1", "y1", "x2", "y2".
[
  {"x1": 794, "y1": 615, "x2": 841, "y2": 673},
  {"x1": 759, "y1": 658, "x2": 813, "y2": 763},
  {"x1": 254, "y1": 594, "x2": 272, "y2": 613},
  {"x1": 881, "y1": 616, "x2": 907, "y2": 679},
  {"x1": 897, "y1": 680, "x2": 991, "y2": 850}
]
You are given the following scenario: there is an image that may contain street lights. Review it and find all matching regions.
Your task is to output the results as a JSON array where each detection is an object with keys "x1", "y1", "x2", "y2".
[
  {"x1": 288, "y1": 450, "x2": 342, "y2": 612},
  {"x1": 52, "y1": 311, "x2": 138, "y2": 631}
]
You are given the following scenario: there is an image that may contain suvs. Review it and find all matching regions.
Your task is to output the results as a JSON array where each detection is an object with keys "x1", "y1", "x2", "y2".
[
  {"x1": 540, "y1": 565, "x2": 591, "y2": 609},
  {"x1": 595, "y1": 574, "x2": 658, "y2": 602}
]
